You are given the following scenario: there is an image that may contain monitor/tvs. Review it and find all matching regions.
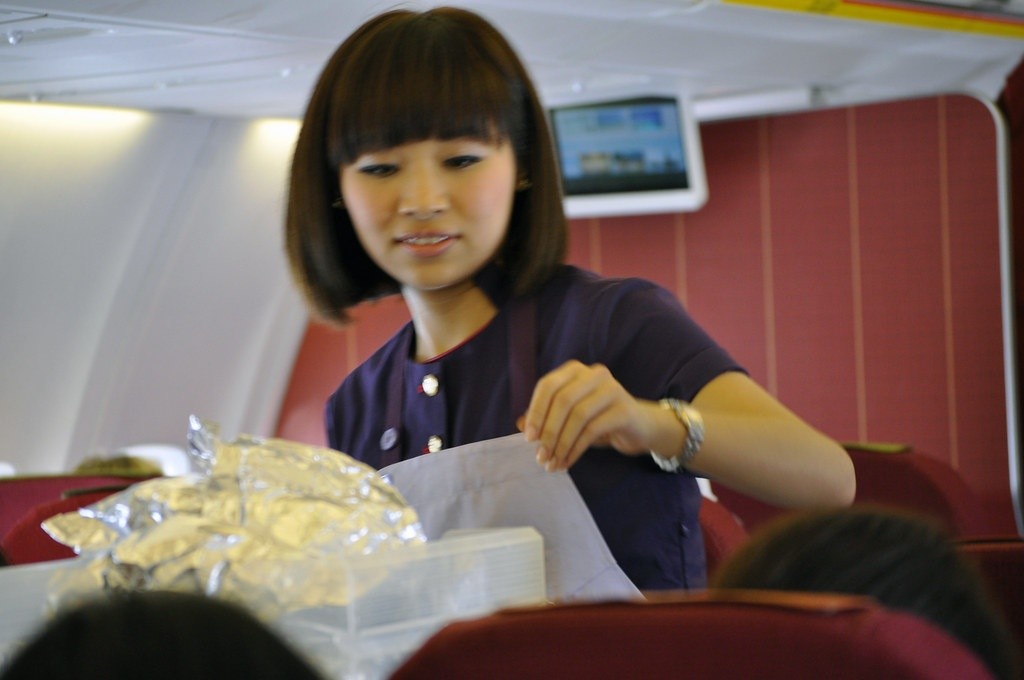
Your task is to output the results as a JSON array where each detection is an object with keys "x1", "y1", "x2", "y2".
[{"x1": 540, "y1": 88, "x2": 713, "y2": 218}]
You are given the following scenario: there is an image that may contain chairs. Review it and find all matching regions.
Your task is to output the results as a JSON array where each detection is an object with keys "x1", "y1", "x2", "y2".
[{"x1": 0, "y1": 440, "x2": 1001, "y2": 680}]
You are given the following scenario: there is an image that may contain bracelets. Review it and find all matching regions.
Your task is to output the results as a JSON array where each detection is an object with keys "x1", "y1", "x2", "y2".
[{"x1": 650, "y1": 398, "x2": 704, "y2": 476}]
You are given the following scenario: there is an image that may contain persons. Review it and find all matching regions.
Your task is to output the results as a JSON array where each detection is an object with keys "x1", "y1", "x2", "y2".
[
  {"x1": 0, "y1": 588, "x2": 324, "y2": 680},
  {"x1": 284, "y1": 6, "x2": 856, "y2": 592},
  {"x1": 711, "y1": 506, "x2": 1024, "y2": 680}
]
